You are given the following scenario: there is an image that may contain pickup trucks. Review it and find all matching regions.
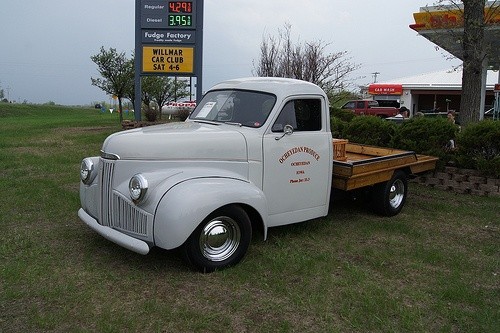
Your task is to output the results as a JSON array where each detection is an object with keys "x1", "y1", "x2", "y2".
[
  {"x1": 340, "y1": 100, "x2": 399, "y2": 120},
  {"x1": 78, "y1": 78, "x2": 441, "y2": 275}
]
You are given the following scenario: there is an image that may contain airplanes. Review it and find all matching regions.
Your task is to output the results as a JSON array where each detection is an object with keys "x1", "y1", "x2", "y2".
[{"x1": 150, "y1": 100, "x2": 196, "y2": 120}]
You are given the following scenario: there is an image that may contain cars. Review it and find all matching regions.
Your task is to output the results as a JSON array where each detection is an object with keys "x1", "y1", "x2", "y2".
[{"x1": 95, "y1": 104, "x2": 102, "y2": 109}]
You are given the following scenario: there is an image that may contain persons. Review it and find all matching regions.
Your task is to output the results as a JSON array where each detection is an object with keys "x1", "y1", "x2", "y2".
[
  {"x1": 261, "y1": 98, "x2": 292, "y2": 132},
  {"x1": 397, "y1": 105, "x2": 466, "y2": 150}
]
